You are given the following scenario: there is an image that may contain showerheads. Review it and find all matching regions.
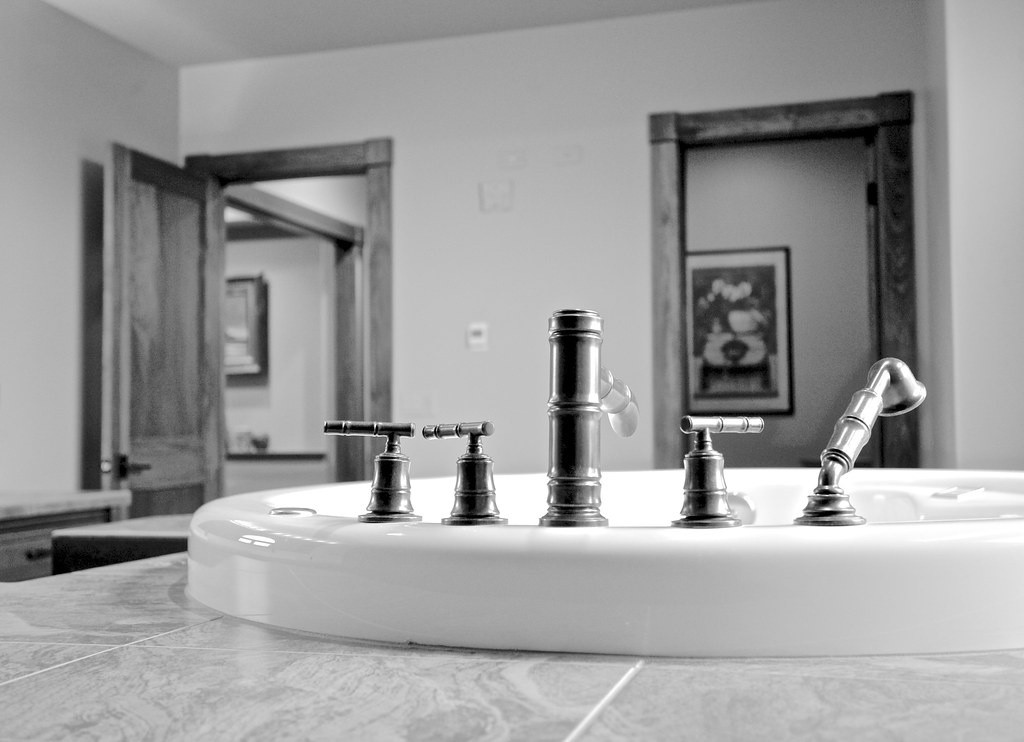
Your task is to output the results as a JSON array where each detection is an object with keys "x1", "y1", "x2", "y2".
[{"x1": 809, "y1": 355, "x2": 928, "y2": 494}]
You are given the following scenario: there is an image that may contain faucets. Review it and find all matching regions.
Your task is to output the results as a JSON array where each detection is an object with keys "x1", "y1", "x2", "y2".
[{"x1": 538, "y1": 307, "x2": 640, "y2": 526}]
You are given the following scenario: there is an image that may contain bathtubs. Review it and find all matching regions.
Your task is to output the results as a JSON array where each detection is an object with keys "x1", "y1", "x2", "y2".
[{"x1": 186, "y1": 467, "x2": 1024, "y2": 655}]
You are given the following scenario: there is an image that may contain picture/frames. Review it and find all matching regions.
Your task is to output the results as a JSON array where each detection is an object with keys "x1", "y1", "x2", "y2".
[{"x1": 685, "y1": 247, "x2": 790, "y2": 413}]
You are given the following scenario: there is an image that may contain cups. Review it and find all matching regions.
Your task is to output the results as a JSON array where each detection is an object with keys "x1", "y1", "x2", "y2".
[
  {"x1": 235, "y1": 431, "x2": 252, "y2": 453},
  {"x1": 252, "y1": 435, "x2": 269, "y2": 453}
]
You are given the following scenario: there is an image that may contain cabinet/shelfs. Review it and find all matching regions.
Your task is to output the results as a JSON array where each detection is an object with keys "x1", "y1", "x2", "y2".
[{"x1": 0, "y1": 506, "x2": 109, "y2": 587}]
